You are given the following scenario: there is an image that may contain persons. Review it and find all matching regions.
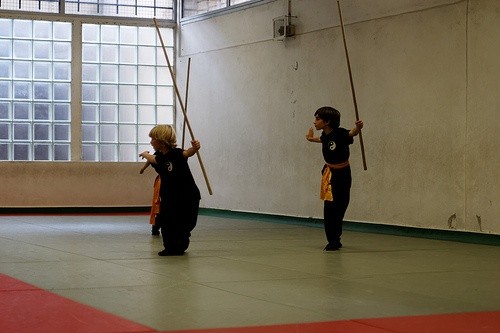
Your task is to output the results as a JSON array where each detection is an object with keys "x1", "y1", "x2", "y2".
[
  {"x1": 139, "y1": 151, "x2": 162, "y2": 236},
  {"x1": 137, "y1": 124, "x2": 202, "y2": 256},
  {"x1": 307, "y1": 106, "x2": 364, "y2": 251}
]
